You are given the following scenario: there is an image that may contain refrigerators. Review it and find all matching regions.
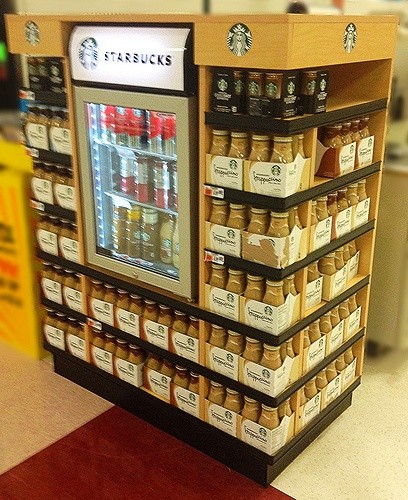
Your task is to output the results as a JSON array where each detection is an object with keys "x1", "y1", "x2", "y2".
[{"x1": 67, "y1": 26, "x2": 200, "y2": 303}]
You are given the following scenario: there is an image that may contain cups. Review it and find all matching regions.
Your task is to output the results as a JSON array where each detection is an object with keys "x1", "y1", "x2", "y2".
[{"x1": 209, "y1": 323, "x2": 296, "y2": 370}]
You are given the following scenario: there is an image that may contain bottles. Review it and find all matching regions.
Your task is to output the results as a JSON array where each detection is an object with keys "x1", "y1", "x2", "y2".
[
  {"x1": 37, "y1": 213, "x2": 78, "y2": 240},
  {"x1": 101, "y1": 103, "x2": 176, "y2": 156},
  {"x1": 208, "y1": 198, "x2": 303, "y2": 240},
  {"x1": 117, "y1": 148, "x2": 178, "y2": 212},
  {"x1": 42, "y1": 262, "x2": 83, "y2": 292},
  {"x1": 22, "y1": 107, "x2": 71, "y2": 154},
  {"x1": 314, "y1": 179, "x2": 368, "y2": 220},
  {"x1": 88, "y1": 279, "x2": 199, "y2": 339},
  {"x1": 209, "y1": 381, "x2": 294, "y2": 432},
  {"x1": 322, "y1": 115, "x2": 370, "y2": 149},
  {"x1": 206, "y1": 262, "x2": 299, "y2": 307},
  {"x1": 44, "y1": 306, "x2": 86, "y2": 340},
  {"x1": 301, "y1": 346, "x2": 355, "y2": 406},
  {"x1": 304, "y1": 291, "x2": 358, "y2": 348},
  {"x1": 91, "y1": 328, "x2": 200, "y2": 395},
  {"x1": 210, "y1": 130, "x2": 307, "y2": 164},
  {"x1": 32, "y1": 160, "x2": 75, "y2": 187},
  {"x1": 110, "y1": 204, "x2": 178, "y2": 267},
  {"x1": 27, "y1": 56, "x2": 64, "y2": 93},
  {"x1": 307, "y1": 238, "x2": 359, "y2": 282}
]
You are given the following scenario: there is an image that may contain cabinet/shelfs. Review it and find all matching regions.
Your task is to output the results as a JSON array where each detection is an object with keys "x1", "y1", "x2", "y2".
[{"x1": 5, "y1": 13, "x2": 397, "y2": 487}]
